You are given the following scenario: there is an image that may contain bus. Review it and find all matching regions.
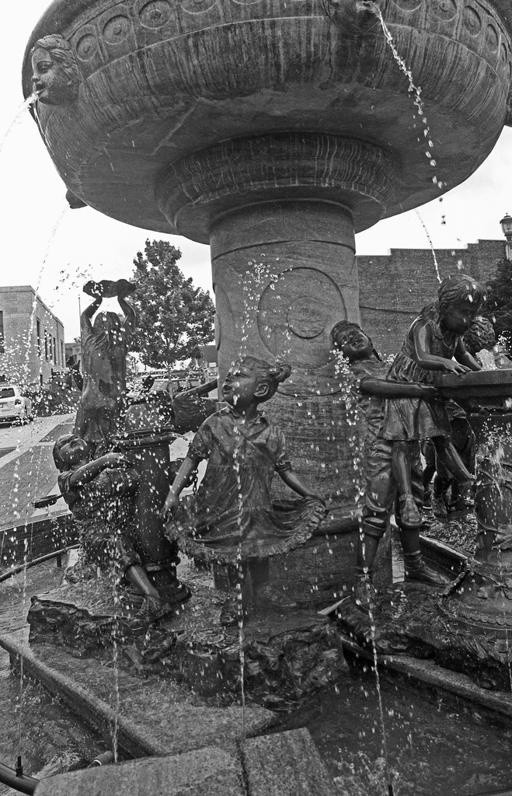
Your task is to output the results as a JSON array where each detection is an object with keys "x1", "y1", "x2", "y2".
[{"x1": 140, "y1": 370, "x2": 167, "y2": 381}]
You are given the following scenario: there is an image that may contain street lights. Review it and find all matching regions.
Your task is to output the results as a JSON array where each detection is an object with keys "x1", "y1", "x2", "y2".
[{"x1": 499, "y1": 213, "x2": 512, "y2": 249}]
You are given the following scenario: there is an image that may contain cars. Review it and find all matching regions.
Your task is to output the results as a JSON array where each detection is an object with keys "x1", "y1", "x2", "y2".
[{"x1": 0, "y1": 387, "x2": 33, "y2": 425}]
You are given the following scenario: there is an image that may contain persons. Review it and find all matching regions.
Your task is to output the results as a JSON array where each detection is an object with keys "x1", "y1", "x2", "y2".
[
  {"x1": 330, "y1": 273, "x2": 496, "y2": 615},
  {"x1": 160, "y1": 356, "x2": 328, "y2": 624},
  {"x1": 52, "y1": 282, "x2": 170, "y2": 621}
]
